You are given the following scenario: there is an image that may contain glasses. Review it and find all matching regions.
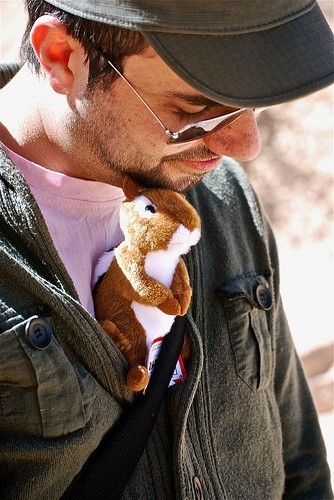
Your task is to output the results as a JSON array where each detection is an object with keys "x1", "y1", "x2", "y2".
[{"x1": 67, "y1": 27, "x2": 271, "y2": 145}]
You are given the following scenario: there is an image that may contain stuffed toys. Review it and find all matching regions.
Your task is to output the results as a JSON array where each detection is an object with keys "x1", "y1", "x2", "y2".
[{"x1": 94, "y1": 173, "x2": 201, "y2": 391}]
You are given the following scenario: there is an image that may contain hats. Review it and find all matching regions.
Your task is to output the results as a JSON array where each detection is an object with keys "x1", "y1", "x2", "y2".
[{"x1": 41, "y1": 0, "x2": 334, "y2": 109}]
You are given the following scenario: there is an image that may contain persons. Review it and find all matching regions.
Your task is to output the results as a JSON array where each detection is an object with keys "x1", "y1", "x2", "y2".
[{"x1": 0, "y1": 0, "x2": 333, "y2": 500}]
